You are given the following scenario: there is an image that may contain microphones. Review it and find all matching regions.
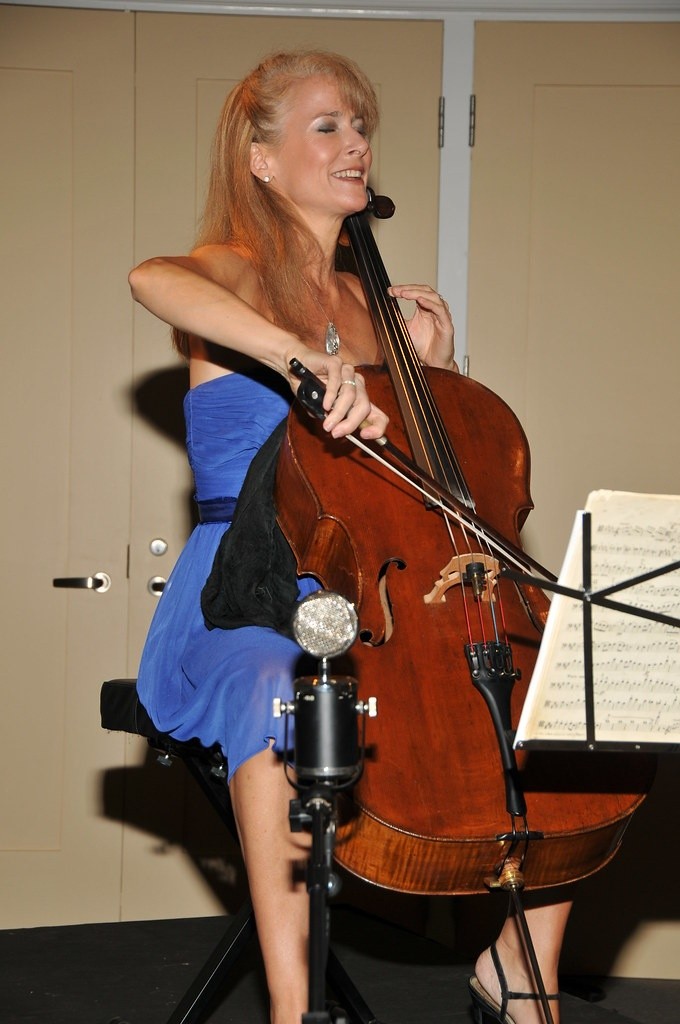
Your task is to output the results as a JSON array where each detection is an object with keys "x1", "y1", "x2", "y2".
[{"x1": 288, "y1": 592, "x2": 360, "y2": 780}]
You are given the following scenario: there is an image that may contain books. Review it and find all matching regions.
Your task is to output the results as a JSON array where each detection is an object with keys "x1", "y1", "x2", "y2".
[{"x1": 513, "y1": 487, "x2": 680, "y2": 752}]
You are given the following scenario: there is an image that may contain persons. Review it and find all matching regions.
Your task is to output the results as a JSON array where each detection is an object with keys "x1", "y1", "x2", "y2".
[{"x1": 128, "y1": 52, "x2": 572, "y2": 1024}]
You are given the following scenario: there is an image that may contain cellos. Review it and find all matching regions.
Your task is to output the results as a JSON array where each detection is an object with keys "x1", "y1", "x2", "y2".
[{"x1": 272, "y1": 169, "x2": 655, "y2": 1024}]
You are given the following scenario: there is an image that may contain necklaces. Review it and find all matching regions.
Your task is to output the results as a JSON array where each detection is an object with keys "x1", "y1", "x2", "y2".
[{"x1": 298, "y1": 270, "x2": 340, "y2": 355}]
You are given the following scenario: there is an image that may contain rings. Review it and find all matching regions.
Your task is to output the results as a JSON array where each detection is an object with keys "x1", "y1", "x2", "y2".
[{"x1": 342, "y1": 380, "x2": 356, "y2": 385}]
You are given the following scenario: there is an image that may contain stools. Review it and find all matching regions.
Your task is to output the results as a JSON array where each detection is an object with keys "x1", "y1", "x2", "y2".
[{"x1": 101, "y1": 679, "x2": 384, "y2": 1024}]
